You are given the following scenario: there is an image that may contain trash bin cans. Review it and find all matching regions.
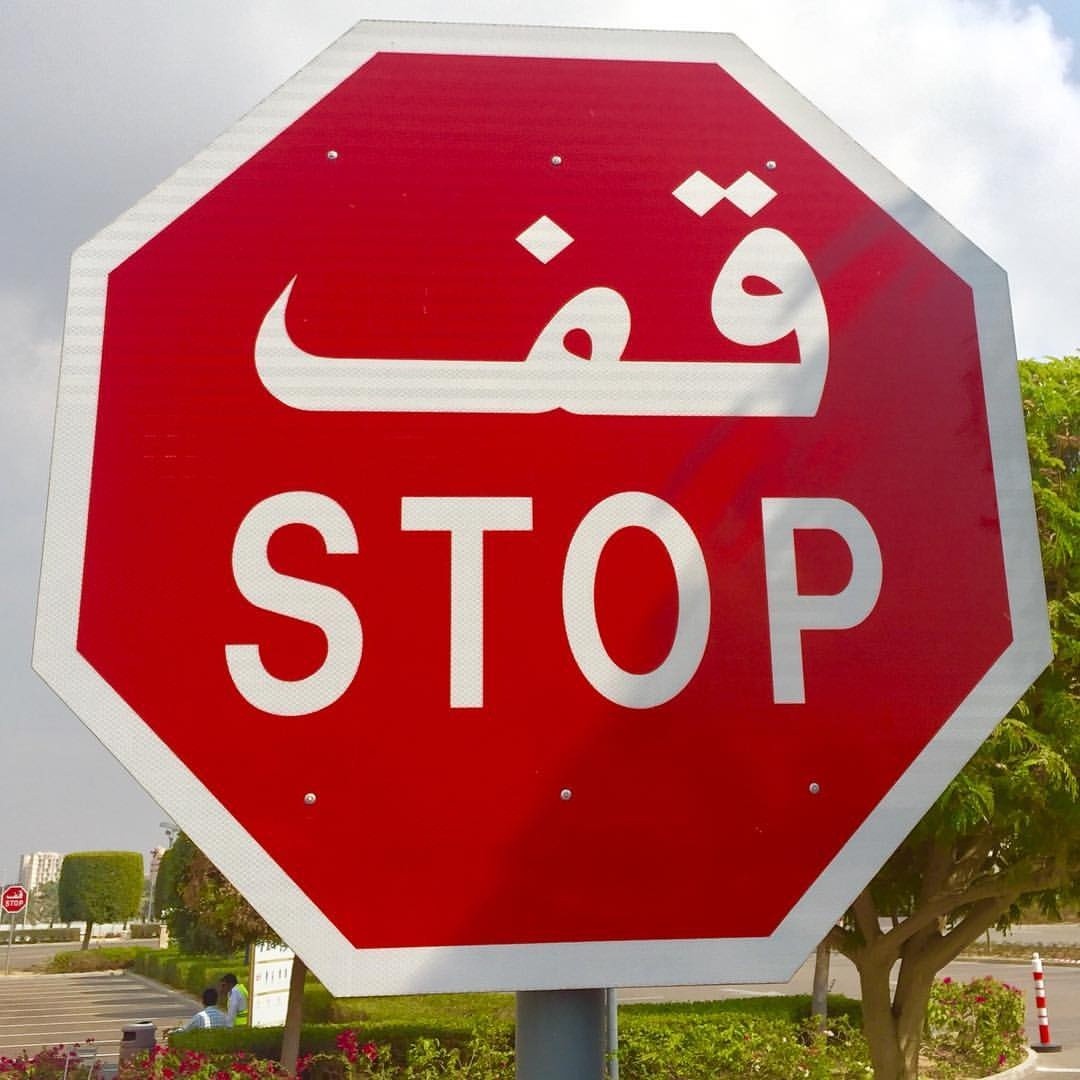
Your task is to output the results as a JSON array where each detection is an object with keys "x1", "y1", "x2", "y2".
[{"x1": 119, "y1": 1021, "x2": 158, "y2": 1058}]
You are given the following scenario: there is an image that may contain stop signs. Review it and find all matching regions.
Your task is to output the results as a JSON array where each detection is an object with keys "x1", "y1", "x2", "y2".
[{"x1": 19, "y1": 15, "x2": 1065, "y2": 1007}]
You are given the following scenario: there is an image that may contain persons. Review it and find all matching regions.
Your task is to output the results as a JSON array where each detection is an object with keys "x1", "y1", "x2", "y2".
[
  {"x1": 218, "y1": 974, "x2": 250, "y2": 1027},
  {"x1": 182, "y1": 988, "x2": 230, "y2": 1032}
]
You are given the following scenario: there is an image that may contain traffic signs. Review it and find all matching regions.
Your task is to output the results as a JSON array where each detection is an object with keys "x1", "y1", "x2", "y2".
[{"x1": 0, "y1": 885, "x2": 29, "y2": 913}]
